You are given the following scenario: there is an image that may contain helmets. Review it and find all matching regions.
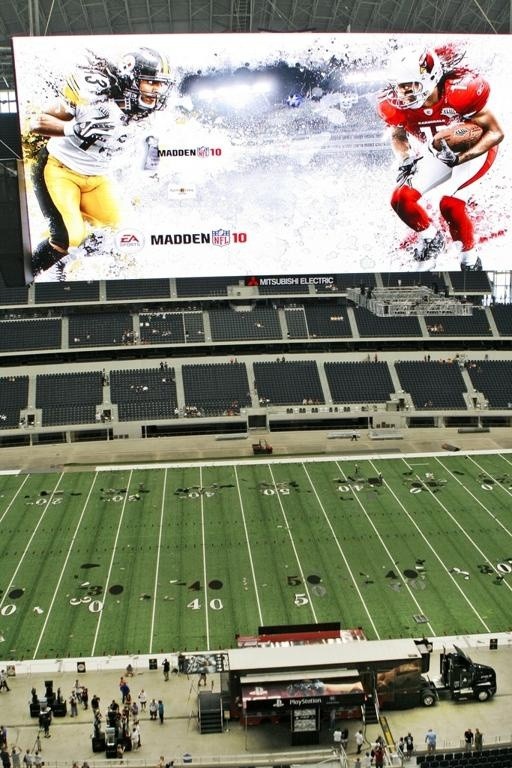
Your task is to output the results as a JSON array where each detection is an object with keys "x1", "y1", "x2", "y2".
[
  {"x1": 385, "y1": 45, "x2": 445, "y2": 111},
  {"x1": 110, "y1": 48, "x2": 173, "y2": 119}
]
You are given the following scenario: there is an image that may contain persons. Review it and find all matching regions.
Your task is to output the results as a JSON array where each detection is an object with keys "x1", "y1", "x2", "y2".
[
  {"x1": 1, "y1": 670, "x2": 10, "y2": 692},
  {"x1": 126, "y1": 664, "x2": 133, "y2": 676},
  {"x1": 30, "y1": 44, "x2": 173, "y2": 278},
  {"x1": 37, "y1": 677, "x2": 146, "y2": 758},
  {"x1": 1, "y1": 726, "x2": 7, "y2": 749},
  {"x1": 362, "y1": 736, "x2": 386, "y2": 767},
  {"x1": 1, "y1": 745, "x2": 43, "y2": 767},
  {"x1": 464, "y1": 728, "x2": 482, "y2": 753},
  {"x1": 198, "y1": 665, "x2": 209, "y2": 687},
  {"x1": 162, "y1": 659, "x2": 170, "y2": 681},
  {"x1": 398, "y1": 732, "x2": 413, "y2": 759},
  {"x1": 332, "y1": 728, "x2": 349, "y2": 749},
  {"x1": 0, "y1": 306, "x2": 511, "y2": 428},
  {"x1": 149, "y1": 699, "x2": 163, "y2": 724},
  {"x1": 376, "y1": 40, "x2": 503, "y2": 272},
  {"x1": 355, "y1": 730, "x2": 363, "y2": 754},
  {"x1": 425, "y1": 728, "x2": 437, "y2": 754},
  {"x1": 178, "y1": 653, "x2": 185, "y2": 673}
]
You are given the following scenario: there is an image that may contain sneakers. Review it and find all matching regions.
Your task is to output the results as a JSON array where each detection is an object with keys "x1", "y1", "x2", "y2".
[
  {"x1": 412, "y1": 233, "x2": 444, "y2": 271},
  {"x1": 460, "y1": 259, "x2": 482, "y2": 271},
  {"x1": 47, "y1": 260, "x2": 68, "y2": 281}
]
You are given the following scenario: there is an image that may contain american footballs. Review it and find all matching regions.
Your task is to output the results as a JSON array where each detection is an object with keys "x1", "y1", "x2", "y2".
[
  {"x1": 29, "y1": 97, "x2": 75, "y2": 131},
  {"x1": 434, "y1": 123, "x2": 483, "y2": 152}
]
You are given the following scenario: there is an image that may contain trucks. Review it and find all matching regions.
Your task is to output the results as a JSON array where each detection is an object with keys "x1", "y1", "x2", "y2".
[{"x1": 378, "y1": 640, "x2": 497, "y2": 712}]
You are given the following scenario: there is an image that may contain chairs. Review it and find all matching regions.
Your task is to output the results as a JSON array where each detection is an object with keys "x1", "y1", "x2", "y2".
[
  {"x1": 414, "y1": 739, "x2": 512, "y2": 767},
  {"x1": 0, "y1": 266, "x2": 510, "y2": 457}
]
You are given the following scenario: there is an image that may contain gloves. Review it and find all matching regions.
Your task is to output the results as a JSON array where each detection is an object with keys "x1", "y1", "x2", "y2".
[
  {"x1": 67, "y1": 107, "x2": 117, "y2": 152},
  {"x1": 395, "y1": 155, "x2": 423, "y2": 189},
  {"x1": 428, "y1": 139, "x2": 459, "y2": 167}
]
